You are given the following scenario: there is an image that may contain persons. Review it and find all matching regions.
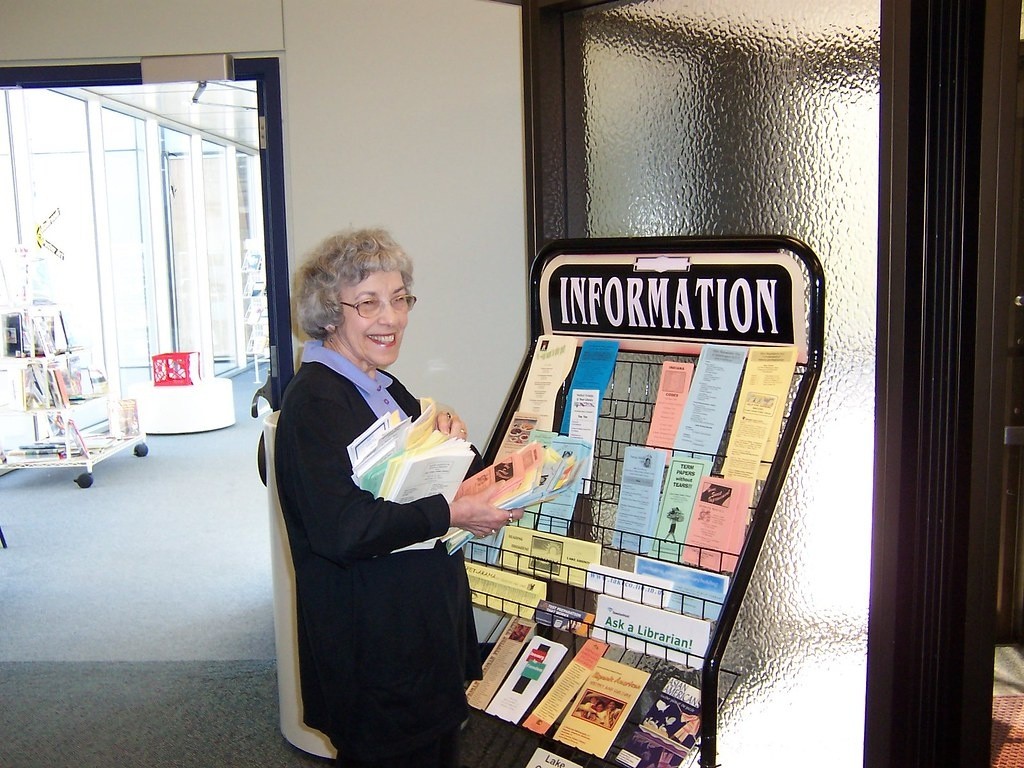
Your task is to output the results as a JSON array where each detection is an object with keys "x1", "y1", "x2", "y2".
[{"x1": 273, "y1": 226, "x2": 525, "y2": 768}]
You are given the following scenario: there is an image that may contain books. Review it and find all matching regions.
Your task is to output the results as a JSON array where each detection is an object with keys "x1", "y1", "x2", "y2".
[
  {"x1": 346, "y1": 396, "x2": 476, "y2": 556},
  {"x1": 1, "y1": 307, "x2": 141, "y2": 464},
  {"x1": 551, "y1": 594, "x2": 713, "y2": 768}
]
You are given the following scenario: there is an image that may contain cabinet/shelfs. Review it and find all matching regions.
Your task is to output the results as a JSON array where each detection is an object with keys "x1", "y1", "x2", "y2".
[
  {"x1": 433, "y1": 232, "x2": 826, "y2": 768},
  {"x1": 1, "y1": 246, "x2": 149, "y2": 488}
]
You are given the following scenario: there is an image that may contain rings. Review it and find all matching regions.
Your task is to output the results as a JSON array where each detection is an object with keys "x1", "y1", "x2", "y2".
[
  {"x1": 483, "y1": 534, "x2": 484, "y2": 538},
  {"x1": 441, "y1": 412, "x2": 451, "y2": 419},
  {"x1": 461, "y1": 428, "x2": 467, "y2": 433},
  {"x1": 491, "y1": 528, "x2": 496, "y2": 535},
  {"x1": 509, "y1": 509, "x2": 513, "y2": 523}
]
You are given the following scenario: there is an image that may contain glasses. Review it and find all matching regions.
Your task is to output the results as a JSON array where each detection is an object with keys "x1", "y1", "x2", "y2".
[{"x1": 334, "y1": 295, "x2": 417, "y2": 319}]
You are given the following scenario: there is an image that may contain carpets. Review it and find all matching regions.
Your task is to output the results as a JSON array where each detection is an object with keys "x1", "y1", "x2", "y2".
[{"x1": 0, "y1": 659, "x2": 323, "y2": 768}]
